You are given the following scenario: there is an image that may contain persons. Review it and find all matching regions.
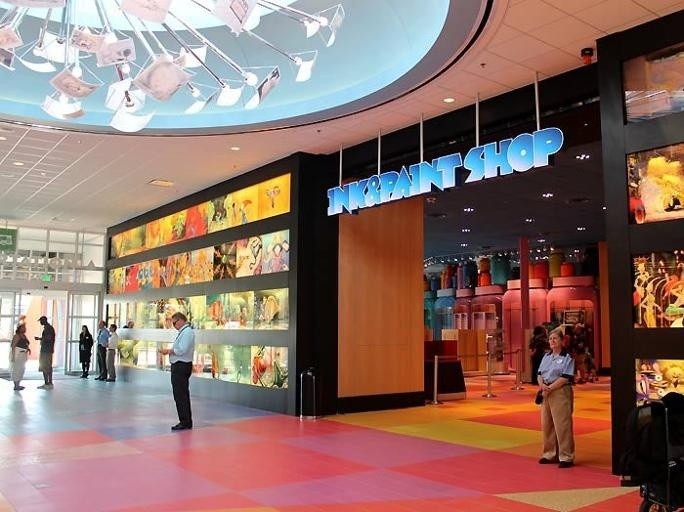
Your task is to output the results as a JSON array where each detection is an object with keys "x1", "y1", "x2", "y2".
[
  {"x1": 78, "y1": 324, "x2": 93, "y2": 379},
  {"x1": 157, "y1": 311, "x2": 196, "y2": 430},
  {"x1": 105, "y1": 323, "x2": 119, "y2": 381},
  {"x1": 123, "y1": 321, "x2": 134, "y2": 328},
  {"x1": 569, "y1": 326, "x2": 587, "y2": 384},
  {"x1": 33, "y1": 316, "x2": 56, "y2": 390},
  {"x1": 536, "y1": 329, "x2": 576, "y2": 467},
  {"x1": 527, "y1": 326, "x2": 545, "y2": 381},
  {"x1": 7, "y1": 320, "x2": 31, "y2": 390},
  {"x1": 94, "y1": 321, "x2": 110, "y2": 380},
  {"x1": 561, "y1": 326, "x2": 573, "y2": 356}
]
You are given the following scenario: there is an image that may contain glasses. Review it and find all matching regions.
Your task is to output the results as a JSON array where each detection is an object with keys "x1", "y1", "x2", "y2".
[{"x1": 172, "y1": 318, "x2": 180, "y2": 326}]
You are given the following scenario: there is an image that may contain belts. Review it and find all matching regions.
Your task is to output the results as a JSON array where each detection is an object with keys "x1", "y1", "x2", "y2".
[{"x1": 108, "y1": 349, "x2": 115, "y2": 350}]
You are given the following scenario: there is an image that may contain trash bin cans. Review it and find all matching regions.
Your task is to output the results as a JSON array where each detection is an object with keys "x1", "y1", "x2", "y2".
[{"x1": 299, "y1": 363, "x2": 323, "y2": 416}]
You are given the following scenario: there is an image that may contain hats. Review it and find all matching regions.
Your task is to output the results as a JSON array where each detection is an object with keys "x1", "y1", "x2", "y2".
[
  {"x1": 37, "y1": 315, "x2": 47, "y2": 321},
  {"x1": 17, "y1": 321, "x2": 25, "y2": 328}
]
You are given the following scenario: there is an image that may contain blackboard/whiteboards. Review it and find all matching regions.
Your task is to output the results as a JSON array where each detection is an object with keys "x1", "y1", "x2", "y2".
[{"x1": 430, "y1": 362, "x2": 467, "y2": 393}]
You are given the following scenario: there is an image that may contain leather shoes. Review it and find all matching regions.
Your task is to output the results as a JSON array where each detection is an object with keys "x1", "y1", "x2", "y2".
[{"x1": 171, "y1": 420, "x2": 193, "y2": 430}]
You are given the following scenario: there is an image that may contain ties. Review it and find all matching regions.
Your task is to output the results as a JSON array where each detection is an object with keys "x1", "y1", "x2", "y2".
[{"x1": 97, "y1": 330, "x2": 102, "y2": 345}]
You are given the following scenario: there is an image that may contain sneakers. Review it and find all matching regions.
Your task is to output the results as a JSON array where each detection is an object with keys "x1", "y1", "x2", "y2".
[
  {"x1": 94, "y1": 375, "x2": 116, "y2": 382},
  {"x1": 558, "y1": 461, "x2": 574, "y2": 468},
  {"x1": 37, "y1": 383, "x2": 54, "y2": 390},
  {"x1": 538, "y1": 458, "x2": 559, "y2": 465},
  {"x1": 14, "y1": 386, "x2": 25, "y2": 391}
]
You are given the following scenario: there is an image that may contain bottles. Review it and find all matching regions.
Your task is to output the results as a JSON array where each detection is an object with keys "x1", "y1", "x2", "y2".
[
  {"x1": 422, "y1": 248, "x2": 597, "y2": 288},
  {"x1": 548, "y1": 276, "x2": 601, "y2": 373},
  {"x1": 502, "y1": 278, "x2": 546, "y2": 375},
  {"x1": 471, "y1": 286, "x2": 503, "y2": 372},
  {"x1": 425, "y1": 289, "x2": 480, "y2": 374}
]
